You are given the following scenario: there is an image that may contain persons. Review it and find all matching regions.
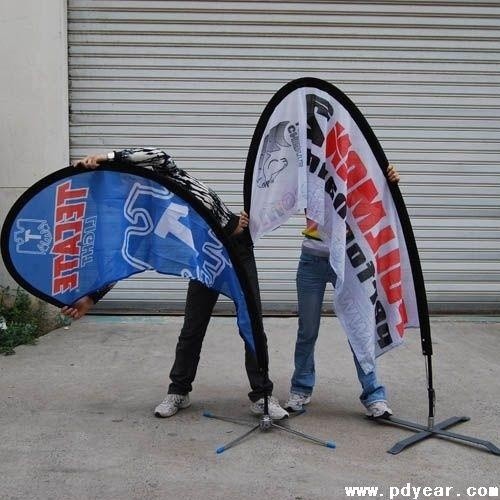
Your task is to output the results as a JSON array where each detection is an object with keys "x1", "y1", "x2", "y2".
[
  {"x1": 57, "y1": 146, "x2": 291, "y2": 424},
  {"x1": 237, "y1": 163, "x2": 401, "y2": 423}
]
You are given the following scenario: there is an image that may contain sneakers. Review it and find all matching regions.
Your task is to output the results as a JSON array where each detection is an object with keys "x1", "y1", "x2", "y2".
[
  {"x1": 250, "y1": 396, "x2": 290, "y2": 420},
  {"x1": 154, "y1": 392, "x2": 191, "y2": 418},
  {"x1": 365, "y1": 400, "x2": 392, "y2": 419},
  {"x1": 283, "y1": 392, "x2": 312, "y2": 411}
]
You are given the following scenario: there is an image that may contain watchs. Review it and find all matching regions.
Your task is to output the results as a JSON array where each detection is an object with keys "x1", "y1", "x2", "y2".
[{"x1": 106, "y1": 151, "x2": 115, "y2": 162}]
